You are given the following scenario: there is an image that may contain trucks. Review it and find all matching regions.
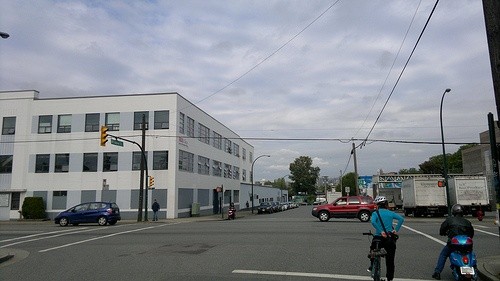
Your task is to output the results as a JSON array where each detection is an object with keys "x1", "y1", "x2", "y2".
[
  {"x1": 398, "y1": 176, "x2": 449, "y2": 218},
  {"x1": 445, "y1": 174, "x2": 491, "y2": 217}
]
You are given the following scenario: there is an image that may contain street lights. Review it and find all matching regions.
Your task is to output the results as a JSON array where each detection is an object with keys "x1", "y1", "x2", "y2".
[
  {"x1": 251, "y1": 155, "x2": 270, "y2": 213},
  {"x1": 439, "y1": 88, "x2": 452, "y2": 216}
]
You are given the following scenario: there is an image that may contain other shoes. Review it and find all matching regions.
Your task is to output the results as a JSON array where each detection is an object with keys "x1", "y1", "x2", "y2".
[
  {"x1": 432, "y1": 273, "x2": 440, "y2": 280},
  {"x1": 367, "y1": 267, "x2": 371, "y2": 273}
]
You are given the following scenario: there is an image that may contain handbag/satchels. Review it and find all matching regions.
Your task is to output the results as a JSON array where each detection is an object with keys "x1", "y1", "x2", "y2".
[{"x1": 388, "y1": 231, "x2": 399, "y2": 241}]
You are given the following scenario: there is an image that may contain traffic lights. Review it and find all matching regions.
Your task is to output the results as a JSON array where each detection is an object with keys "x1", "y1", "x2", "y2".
[
  {"x1": 149, "y1": 176, "x2": 155, "y2": 187},
  {"x1": 100, "y1": 125, "x2": 109, "y2": 146}
]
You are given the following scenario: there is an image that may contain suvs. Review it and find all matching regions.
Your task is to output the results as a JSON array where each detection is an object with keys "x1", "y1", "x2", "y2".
[{"x1": 312, "y1": 195, "x2": 378, "y2": 222}]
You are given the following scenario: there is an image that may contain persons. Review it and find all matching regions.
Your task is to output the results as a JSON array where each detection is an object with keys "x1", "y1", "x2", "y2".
[
  {"x1": 151, "y1": 199, "x2": 160, "y2": 221},
  {"x1": 366, "y1": 196, "x2": 404, "y2": 281},
  {"x1": 228, "y1": 203, "x2": 236, "y2": 219},
  {"x1": 246, "y1": 201, "x2": 249, "y2": 210},
  {"x1": 431, "y1": 204, "x2": 474, "y2": 280}
]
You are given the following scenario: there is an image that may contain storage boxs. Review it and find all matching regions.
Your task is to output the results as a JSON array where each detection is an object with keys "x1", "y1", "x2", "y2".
[{"x1": 452, "y1": 235, "x2": 473, "y2": 255}]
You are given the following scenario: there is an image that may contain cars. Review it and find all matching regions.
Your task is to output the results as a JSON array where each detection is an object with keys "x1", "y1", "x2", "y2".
[
  {"x1": 257, "y1": 201, "x2": 301, "y2": 214},
  {"x1": 54, "y1": 201, "x2": 121, "y2": 227}
]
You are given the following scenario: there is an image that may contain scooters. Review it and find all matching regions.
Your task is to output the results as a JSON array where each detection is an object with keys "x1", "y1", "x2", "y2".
[
  {"x1": 445, "y1": 232, "x2": 479, "y2": 281},
  {"x1": 228, "y1": 208, "x2": 236, "y2": 220}
]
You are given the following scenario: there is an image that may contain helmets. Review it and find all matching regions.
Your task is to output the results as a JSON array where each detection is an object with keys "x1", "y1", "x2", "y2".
[
  {"x1": 374, "y1": 196, "x2": 387, "y2": 205},
  {"x1": 452, "y1": 204, "x2": 463, "y2": 214}
]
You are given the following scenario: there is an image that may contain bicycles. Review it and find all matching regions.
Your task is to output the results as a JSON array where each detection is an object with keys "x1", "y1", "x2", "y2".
[{"x1": 362, "y1": 229, "x2": 385, "y2": 281}]
